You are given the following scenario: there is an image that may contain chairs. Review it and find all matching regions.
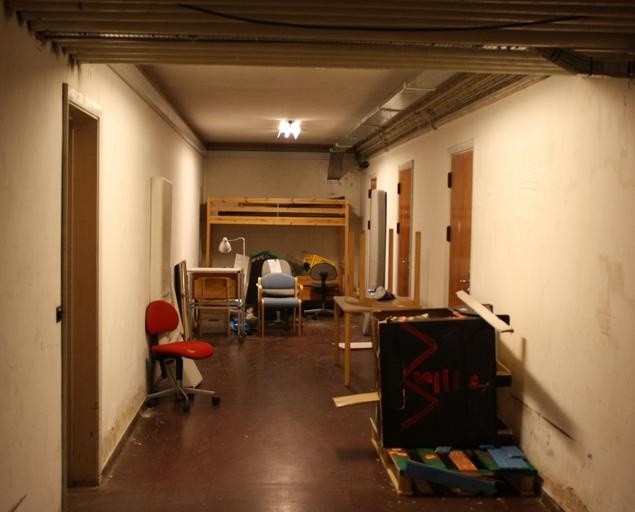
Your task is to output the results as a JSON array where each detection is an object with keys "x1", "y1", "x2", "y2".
[
  {"x1": 255, "y1": 259, "x2": 302, "y2": 338},
  {"x1": 145, "y1": 300, "x2": 220, "y2": 411},
  {"x1": 302, "y1": 263, "x2": 339, "y2": 319}
]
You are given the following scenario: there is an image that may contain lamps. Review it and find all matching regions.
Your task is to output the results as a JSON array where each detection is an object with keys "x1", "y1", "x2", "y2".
[
  {"x1": 276, "y1": 119, "x2": 302, "y2": 140},
  {"x1": 218, "y1": 236, "x2": 246, "y2": 337}
]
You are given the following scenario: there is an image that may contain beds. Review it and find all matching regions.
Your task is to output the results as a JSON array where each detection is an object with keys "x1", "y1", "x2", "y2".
[{"x1": 207, "y1": 197, "x2": 349, "y2": 323}]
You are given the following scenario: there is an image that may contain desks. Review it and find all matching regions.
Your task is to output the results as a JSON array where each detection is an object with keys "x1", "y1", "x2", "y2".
[{"x1": 332, "y1": 295, "x2": 422, "y2": 388}]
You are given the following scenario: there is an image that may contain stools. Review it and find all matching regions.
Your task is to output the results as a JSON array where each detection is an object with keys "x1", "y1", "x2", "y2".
[{"x1": 343, "y1": 228, "x2": 421, "y2": 310}]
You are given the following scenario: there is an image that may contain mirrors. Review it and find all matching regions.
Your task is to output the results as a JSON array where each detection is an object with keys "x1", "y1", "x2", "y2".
[{"x1": 148, "y1": 175, "x2": 173, "y2": 301}]
